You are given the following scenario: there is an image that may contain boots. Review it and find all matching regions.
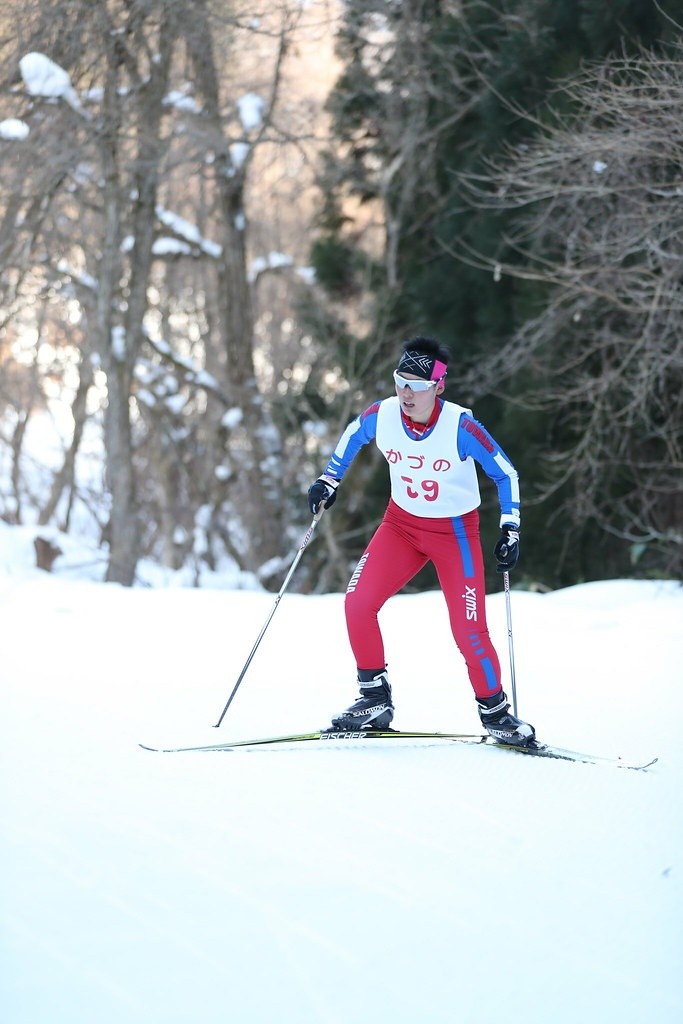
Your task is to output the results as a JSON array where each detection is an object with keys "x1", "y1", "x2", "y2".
[
  {"x1": 331, "y1": 664, "x2": 394, "y2": 730},
  {"x1": 474, "y1": 685, "x2": 535, "y2": 746}
]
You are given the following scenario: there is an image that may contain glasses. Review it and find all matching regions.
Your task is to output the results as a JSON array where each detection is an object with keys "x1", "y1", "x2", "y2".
[{"x1": 393, "y1": 370, "x2": 436, "y2": 392}]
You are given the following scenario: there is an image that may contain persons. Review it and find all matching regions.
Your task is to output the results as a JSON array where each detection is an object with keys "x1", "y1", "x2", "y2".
[{"x1": 308, "y1": 337, "x2": 540, "y2": 746}]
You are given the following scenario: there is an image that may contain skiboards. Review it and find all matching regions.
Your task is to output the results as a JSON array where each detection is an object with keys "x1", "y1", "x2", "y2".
[{"x1": 136, "y1": 728, "x2": 660, "y2": 770}]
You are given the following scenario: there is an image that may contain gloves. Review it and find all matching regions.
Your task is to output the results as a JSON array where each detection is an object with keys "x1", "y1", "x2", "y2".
[
  {"x1": 494, "y1": 522, "x2": 520, "y2": 572},
  {"x1": 307, "y1": 474, "x2": 341, "y2": 515}
]
